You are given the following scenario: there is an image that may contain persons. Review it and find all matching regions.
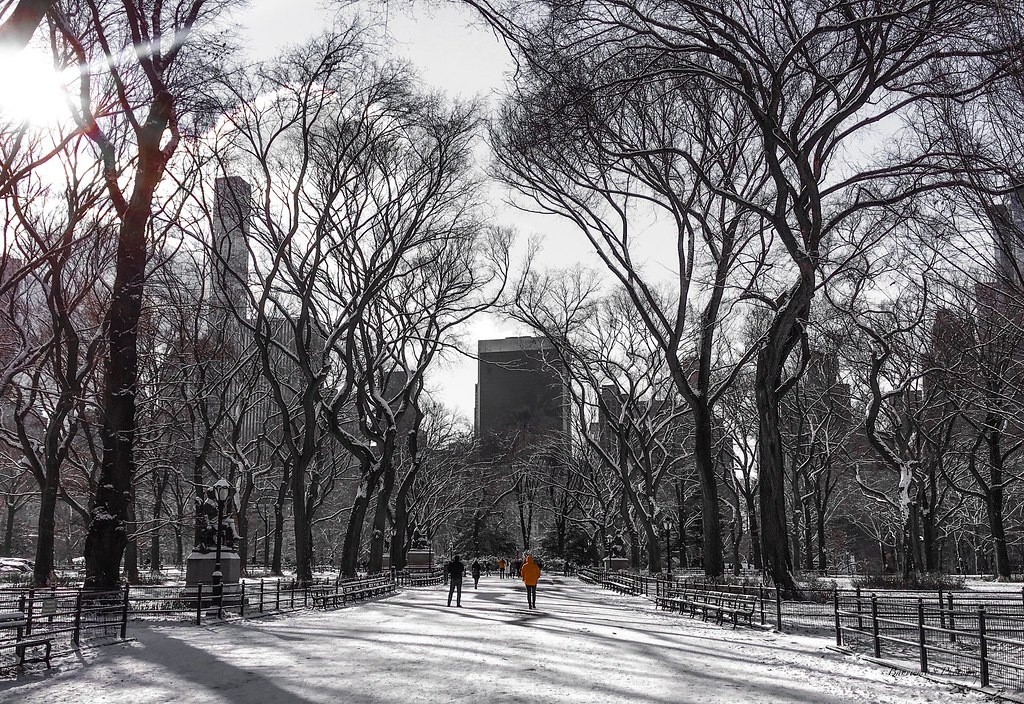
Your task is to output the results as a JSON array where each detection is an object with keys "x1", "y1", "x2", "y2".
[
  {"x1": 521, "y1": 556, "x2": 541, "y2": 609},
  {"x1": 202, "y1": 487, "x2": 244, "y2": 541},
  {"x1": 445, "y1": 556, "x2": 535, "y2": 589},
  {"x1": 446, "y1": 555, "x2": 464, "y2": 607},
  {"x1": 357, "y1": 561, "x2": 369, "y2": 572}
]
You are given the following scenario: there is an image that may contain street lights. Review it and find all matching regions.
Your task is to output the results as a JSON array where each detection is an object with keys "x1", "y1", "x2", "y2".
[
  {"x1": 427, "y1": 538, "x2": 433, "y2": 572},
  {"x1": 205, "y1": 478, "x2": 230, "y2": 617},
  {"x1": 606, "y1": 534, "x2": 614, "y2": 571},
  {"x1": 661, "y1": 515, "x2": 675, "y2": 590},
  {"x1": 449, "y1": 541, "x2": 453, "y2": 561}
]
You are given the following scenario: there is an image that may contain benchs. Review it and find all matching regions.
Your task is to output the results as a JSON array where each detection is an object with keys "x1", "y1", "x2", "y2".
[
  {"x1": 603, "y1": 575, "x2": 635, "y2": 597},
  {"x1": 409, "y1": 570, "x2": 450, "y2": 587},
  {"x1": 655, "y1": 587, "x2": 758, "y2": 630},
  {"x1": 578, "y1": 570, "x2": 595, "y2": 584},
  {"x1": 309, "y1": 577, "x2": 395, "y2": 610},
  {"x1": 0, "y1": 612, "x2": 55, "y2": 669}
]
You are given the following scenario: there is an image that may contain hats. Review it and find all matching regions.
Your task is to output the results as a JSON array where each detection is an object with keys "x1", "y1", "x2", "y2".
[{"x1": 454, "y1": 556, "x2": 460, "y2": 559}]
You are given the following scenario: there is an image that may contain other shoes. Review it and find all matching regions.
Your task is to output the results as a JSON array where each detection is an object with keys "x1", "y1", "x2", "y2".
[
  {"x1": 475, "y1": 585, "x2": 478, "y2": 590},
  {"x1": 529, "y1": 606, "x2": 532, "y2": 609},
  {"x1": 533, "y1": 605, "x2": 536, "y2": 608},
  {"x1": 456, "y1": 604, "x2": 462, "y2": 607},
  {"x1": 446, "y1": 605, "x2": 450, "y2": 607}
]
